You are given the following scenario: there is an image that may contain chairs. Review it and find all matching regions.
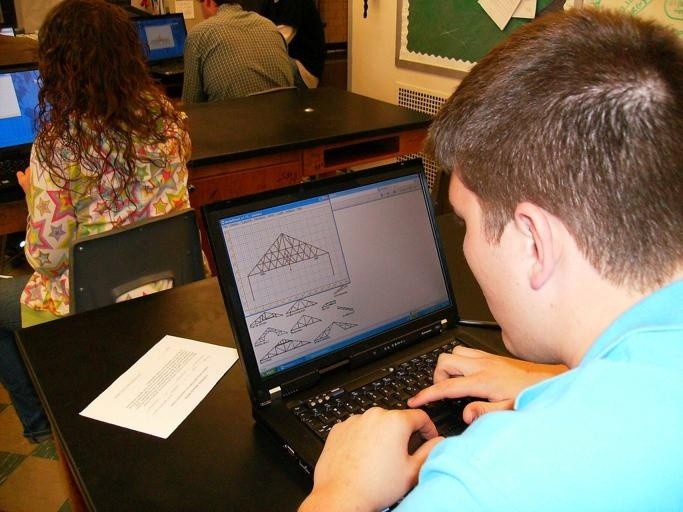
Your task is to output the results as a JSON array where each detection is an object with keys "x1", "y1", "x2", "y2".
[{"x1": 69, "y1": 208, "x2": 206, "y2": 314}]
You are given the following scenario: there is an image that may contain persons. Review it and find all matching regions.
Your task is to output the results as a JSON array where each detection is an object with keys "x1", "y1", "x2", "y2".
[
  {"x1": 108, "y1": 0, "x2": 326, "y2": 103},
  {"x1": 0, "y1": 0, "x2": 212, "y2": 444},
  {"x1": 298, "y1": 9, "x2": 683, "y2": 512}
]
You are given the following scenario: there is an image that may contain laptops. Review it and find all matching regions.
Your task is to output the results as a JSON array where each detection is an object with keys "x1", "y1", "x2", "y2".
[
  {"x1": 130, "y1": 13, "x2": 187, "y2": 78},
  {"x1": 0, "y1": 61, "x2": 57, "y2": 189},
  {"x1": 0, "y1": 25, "x2": 16, "y2": 37},
  {"x1": 200, "y1": 157, "x2": 502, "y2": 512}
]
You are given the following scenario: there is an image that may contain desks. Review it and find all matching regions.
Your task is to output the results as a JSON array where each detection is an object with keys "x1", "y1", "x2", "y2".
[
  {"x1": 15, "y1": 212, "x2": 565, "y2": 507},
  {"x1": 3, "y1": 87, "x2": 437, "y2": 277}
]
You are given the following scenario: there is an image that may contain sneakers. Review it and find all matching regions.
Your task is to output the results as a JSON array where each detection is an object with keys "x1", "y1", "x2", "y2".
[{"x1": 23, "y1": 414, "x2": 53, "y2": 444}]
